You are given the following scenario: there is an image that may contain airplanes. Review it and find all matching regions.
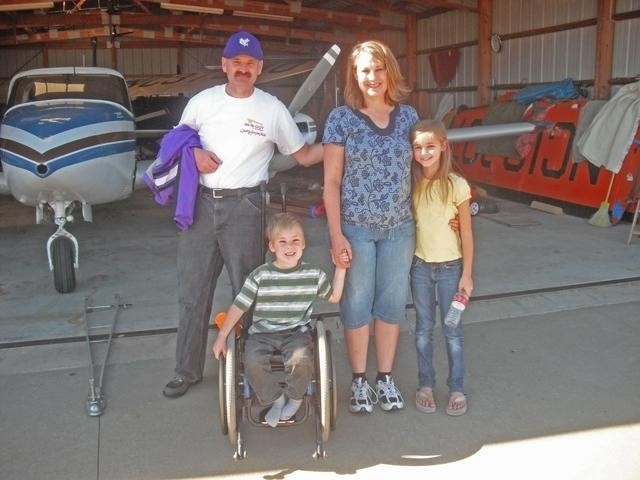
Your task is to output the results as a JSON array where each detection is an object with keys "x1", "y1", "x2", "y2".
[{"x1": 1, "y1": 42, "x2": 537, "y2": 294}]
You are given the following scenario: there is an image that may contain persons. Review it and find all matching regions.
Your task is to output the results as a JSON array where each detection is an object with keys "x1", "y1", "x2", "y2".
[
  {"x1": 323, "y1": 40, "x2": 459, "y2": 415},
  {"x1": 161, "y1": 33, "x2": 323, "y2": 400},
  {"x1": 410, "y1": 119, "x2": 474, "y2": 416},
  {"x1": 212, "y1": 214, "x2": 351, "y2": 426}
]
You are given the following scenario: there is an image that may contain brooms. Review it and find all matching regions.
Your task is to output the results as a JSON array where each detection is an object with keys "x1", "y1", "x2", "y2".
[{"x1": 589, "y1": 171, "x2": 615, "y2": 227}]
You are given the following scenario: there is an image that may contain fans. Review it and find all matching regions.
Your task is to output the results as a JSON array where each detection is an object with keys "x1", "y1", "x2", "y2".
[
  {"x1": 104, "y1": 25, "x2": 132, "y2": 39},
  {"x1": 100, "y1": 0, "x2": 132, "y2": 15}
]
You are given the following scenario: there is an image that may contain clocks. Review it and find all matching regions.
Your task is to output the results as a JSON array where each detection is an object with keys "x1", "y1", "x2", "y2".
[{"x1": 491, "y1": 33, "x2": 501, "y2": 53}]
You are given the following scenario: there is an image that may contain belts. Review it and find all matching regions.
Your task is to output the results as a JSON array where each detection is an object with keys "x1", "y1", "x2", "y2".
[{"x1": 198, "y1": 183, "x2": 271, "y2": 198}]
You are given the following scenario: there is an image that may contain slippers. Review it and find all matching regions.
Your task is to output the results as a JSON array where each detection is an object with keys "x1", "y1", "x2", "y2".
[
  {"x1": 444, "y1": 394, "x2": 468, "y2": 416},
  {"x1": 415, "y1": 388, "x2": 437, "y2": 413}
]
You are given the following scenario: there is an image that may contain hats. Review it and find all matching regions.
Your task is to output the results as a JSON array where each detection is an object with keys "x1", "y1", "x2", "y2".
[{"x1": 221, "y1": 30, "x2": 263, "y2": 61}]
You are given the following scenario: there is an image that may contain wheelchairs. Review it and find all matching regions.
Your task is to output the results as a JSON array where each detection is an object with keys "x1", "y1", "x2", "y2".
[{"x1": 217, "y1": 178, "x2": 336, "y2": 463}]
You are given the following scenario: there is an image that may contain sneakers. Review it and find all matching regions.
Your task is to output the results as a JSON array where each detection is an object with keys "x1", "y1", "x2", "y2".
[
  {"x1": 349, "y1": 377, "x2": 375, "y2": 414},
  {"x1": 161, "y1": 371, "x2": 201, "y2": 399},
  {"x1": 375, "y1": 374, "x2": 405, "y2": 413}
]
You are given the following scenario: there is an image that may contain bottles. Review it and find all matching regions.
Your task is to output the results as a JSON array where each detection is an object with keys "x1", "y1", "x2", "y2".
[{"x1": 443, "y1": 290, "x2": 471, "y2": 329}]
